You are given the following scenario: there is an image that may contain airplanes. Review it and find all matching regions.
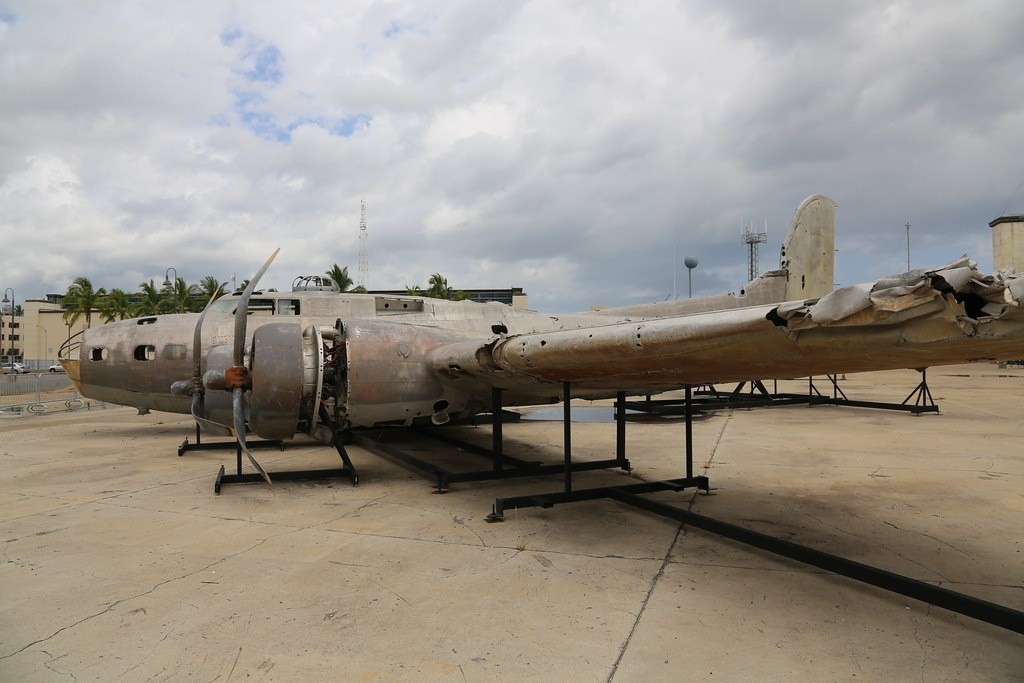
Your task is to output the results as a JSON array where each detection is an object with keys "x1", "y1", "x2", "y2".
[{"x1": 56, "y1": 197, "x2": 1023, "y2": 441}]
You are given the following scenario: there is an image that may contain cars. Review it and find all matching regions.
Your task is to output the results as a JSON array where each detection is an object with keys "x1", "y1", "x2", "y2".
[
  {"x1": 1, "y1": 363, "x2": 30, "y2": 374},
  {"x1": 49, "y1": 362, "x2": 66, "y2": 372}
]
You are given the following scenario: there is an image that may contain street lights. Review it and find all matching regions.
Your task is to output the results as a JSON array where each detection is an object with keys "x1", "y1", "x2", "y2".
[
  {"x1": 65, "y1": 316, "x2": 70, "y2": 359},
  {"x1": 36, "y1": 323, "x2": 47, "y2": 369},
  {"x1": 163, "y1": 268, "x2": 177, "y2": 312},
  {"x1": 2, "y1": 287, "x2": 14, "y2": 373},
  {"x1": 684, "y1": 253, "x2": 700, "y2": 297},
  {"x1": 231, "y1": 272, "x2": 236, "y2": 291}
]
